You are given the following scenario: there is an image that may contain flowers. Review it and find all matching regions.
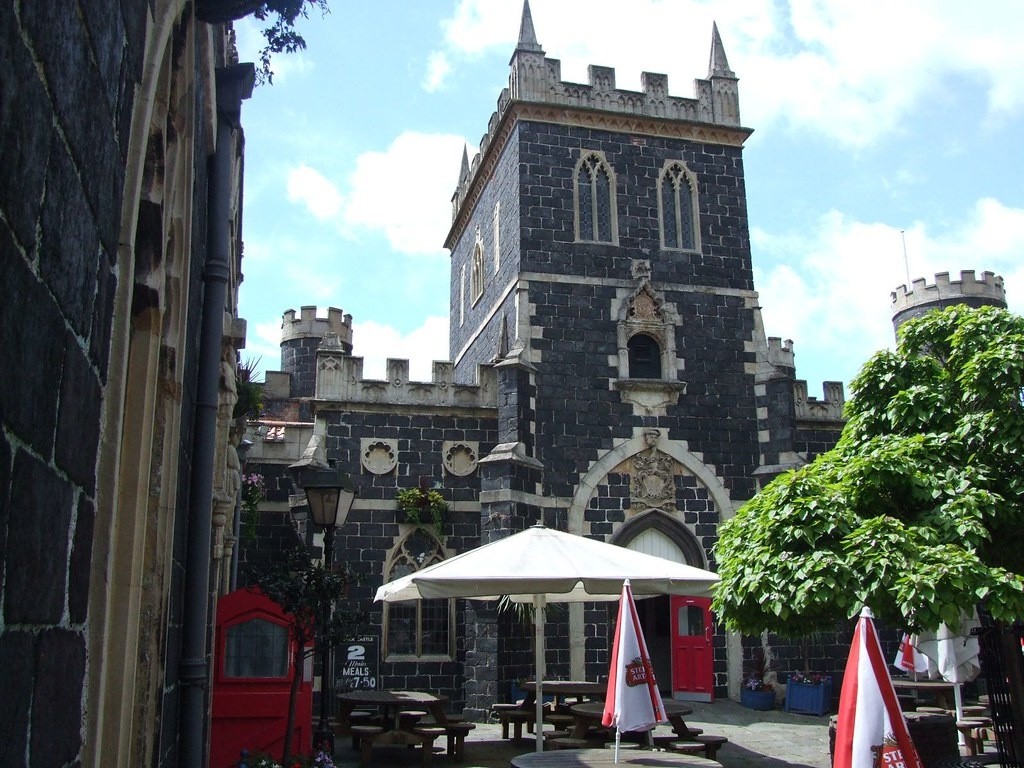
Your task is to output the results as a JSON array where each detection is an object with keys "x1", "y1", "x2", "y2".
[
  {"x1": 738, "y1": 650, "x2": 774, "y2": 692},
  {"x1": 790, "y1": 670, "x2": 830, "y2": 687}
]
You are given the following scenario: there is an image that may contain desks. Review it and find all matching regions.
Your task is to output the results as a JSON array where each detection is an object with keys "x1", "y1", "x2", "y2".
[
  {"x1": 335, "y1": 688, "x2": 450, "y2": 726},
  {"x1": 511, "y1": 749, "x2": 723, "y2": 768},
  {"x1": 892, "y1": 679, "x2": 964, "y2": 690},
  {"x1": 570, "y1": 702, "x2": 693, "y2": 744},
  {"x1": 520, "y1": 680, "x2": 608, "y2": 709}
]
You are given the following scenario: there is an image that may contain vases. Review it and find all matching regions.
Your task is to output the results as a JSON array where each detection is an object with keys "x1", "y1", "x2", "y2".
[
  {"x1": 785, "y1": 679, "x2": 833, "y2": 717},
  {"x1": 740, "y1": 688, "x2": 776, "y2": 711}
]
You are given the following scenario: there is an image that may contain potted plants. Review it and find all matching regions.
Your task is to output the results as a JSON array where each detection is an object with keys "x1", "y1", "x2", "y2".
[{"x1": 395, "y1": 488, "x2": 450, "y2": 556}]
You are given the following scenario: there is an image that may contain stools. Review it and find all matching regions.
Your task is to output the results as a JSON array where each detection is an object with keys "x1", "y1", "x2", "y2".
[
  {"x1": 333, "y1": 699, "x2": 727, "y2": 768},
  {"x1": 898, "y1": 694, "x2": 1009, "y2": 757}
]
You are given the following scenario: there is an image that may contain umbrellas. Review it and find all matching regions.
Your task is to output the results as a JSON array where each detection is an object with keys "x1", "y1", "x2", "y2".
[
  {"x1": 834, "y1": 599, "x2": 982, "y2": 768},
  {"x1": 372, "y1": 524, "x2": 721, "y2": 765}
]
